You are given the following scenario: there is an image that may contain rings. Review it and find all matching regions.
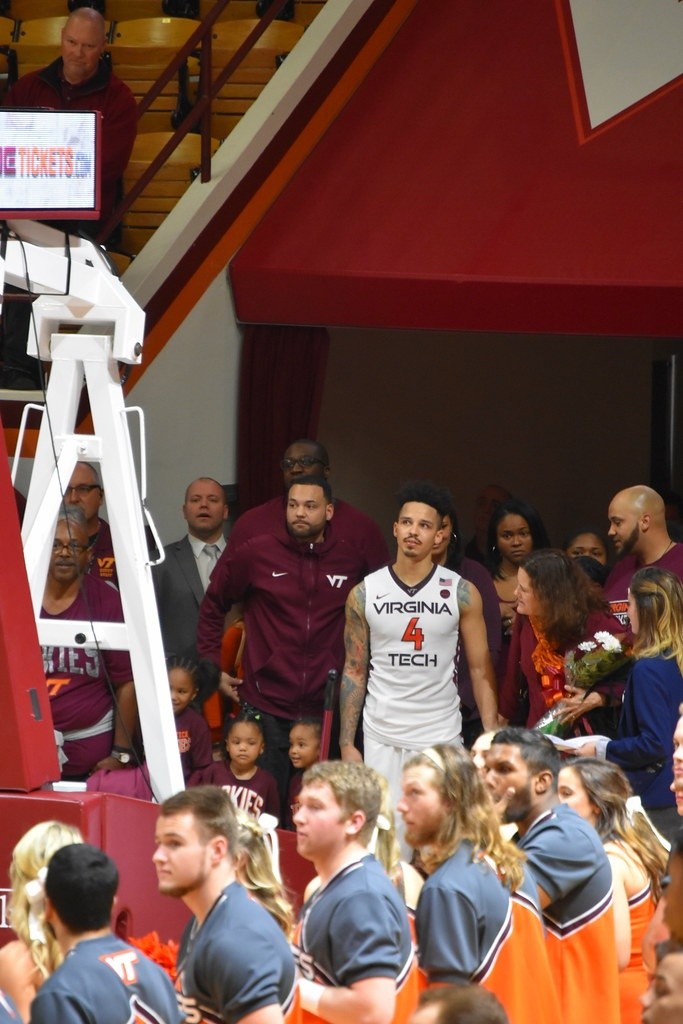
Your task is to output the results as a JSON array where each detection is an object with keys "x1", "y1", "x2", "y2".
[
  {"x1": 573, "y1": 716, "x2": 576, "y2": 719},
  {"x1": 508, "y1": 620, "x2": 513, "y2": 625}
]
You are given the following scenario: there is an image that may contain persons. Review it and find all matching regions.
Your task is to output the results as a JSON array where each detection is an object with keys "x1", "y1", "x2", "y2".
[
  {"x1": 1, "y1": 8, "x2": 137, "y2": 253},
  {"x1": 1, "y1": 438, "x2": 683, "y2": 1024}
]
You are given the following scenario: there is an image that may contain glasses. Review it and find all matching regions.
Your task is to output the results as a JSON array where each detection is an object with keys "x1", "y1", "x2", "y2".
[
  {"x1": 282, "y1": 456, "x2": 324, "y2": 471},
  {"x1": 54, "y1": 543, "x2": 87, "y2": 556},
  {"x1": 66, "y1": 484, "x2": 99, "y2": 495}
]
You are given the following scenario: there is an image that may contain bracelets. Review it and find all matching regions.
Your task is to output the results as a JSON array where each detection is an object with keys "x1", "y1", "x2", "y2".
[
  {"x1": 112, "y1": 745, "x2": 133, "y2": 755},
  {"x1": 298, "y1": 978, "x2": 325, "y2": 1017}
]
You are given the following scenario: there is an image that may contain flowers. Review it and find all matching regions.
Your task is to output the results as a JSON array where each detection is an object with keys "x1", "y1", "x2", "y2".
[{"x1": 535, "y1": 628, "x2": 634, "y2": 737}]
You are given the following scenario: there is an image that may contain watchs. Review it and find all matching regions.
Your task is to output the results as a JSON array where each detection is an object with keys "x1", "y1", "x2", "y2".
[{"x1": 110, "y1": 751, "x2": 131, "y2": 764}]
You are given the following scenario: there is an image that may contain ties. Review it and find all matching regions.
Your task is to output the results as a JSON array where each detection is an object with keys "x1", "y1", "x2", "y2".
[{"x1": 203, "y1": 544, "x2": 219, "y2": 580}]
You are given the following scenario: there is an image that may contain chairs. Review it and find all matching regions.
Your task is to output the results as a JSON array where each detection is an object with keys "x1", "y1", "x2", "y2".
[{"x1": 1, "y1": 0, "x2": 326, "y2": 279}]
